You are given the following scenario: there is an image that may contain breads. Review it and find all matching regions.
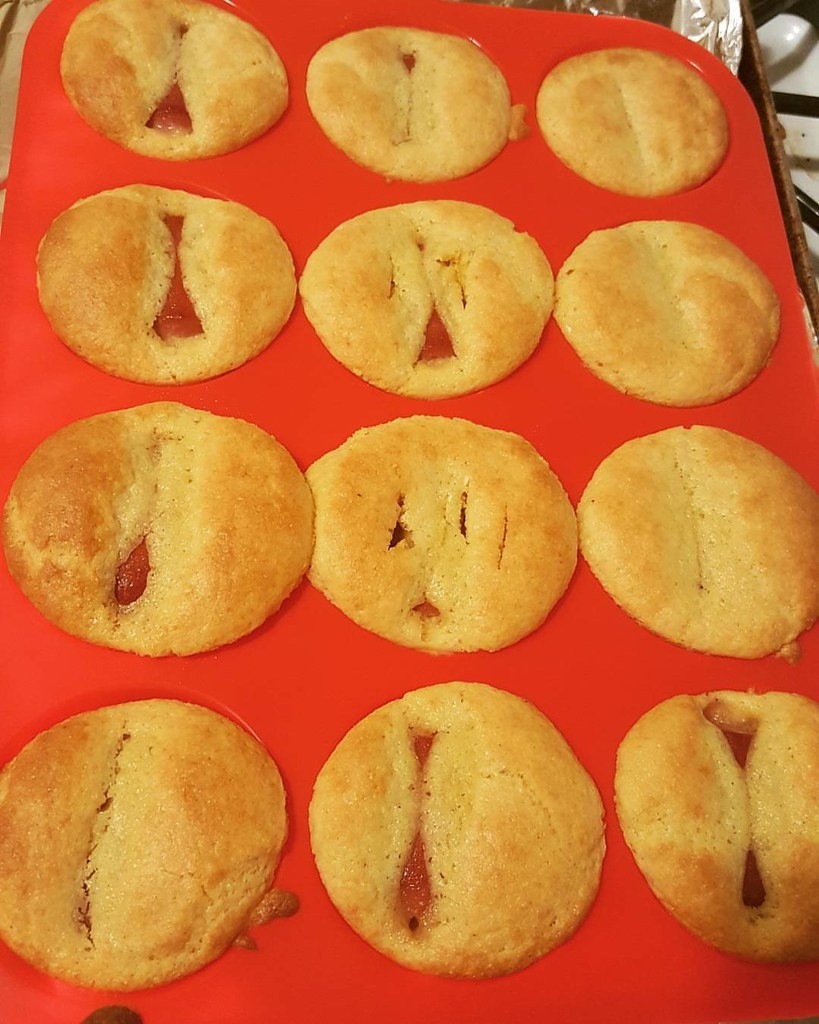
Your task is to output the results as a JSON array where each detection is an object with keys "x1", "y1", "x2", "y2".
[
  {"x1": 613, "y1": 688, "x2": 819, "y2": 966},
  {"x1": 59, "y1": 0, "x2": 289, "y2": 160},
  {"x1": 550, "y1": 218, "x2": 782, "y2": 407},
  {"x1": 5, "y1": 398, "x2": 313, "y2": 659},
  {"x1": 302, "y1": 416, "x2": 580, "y2": 655},
  {"x1": 1, "y1": 697, "x2": 291, "y2": 993},
  {"x1": 306, "y1": 25, "x2": 526, "y2": 184},
  {"x1": 535, "y1": 46, "x2": 729, "y2": 198},
  {"x1": 298, "y1": 198, "x2": 556, "y2": 398},
  {"x1": 309, "y1": 680, "x2": 608, "y2": 977},
  {"x1": 33, "y1": 182, "x2": 298, "y2": 384},
  {"x1": 576, "y1": 424, "x2": 819, "y2": 660}
]
